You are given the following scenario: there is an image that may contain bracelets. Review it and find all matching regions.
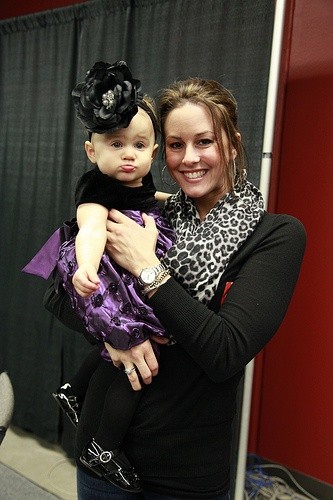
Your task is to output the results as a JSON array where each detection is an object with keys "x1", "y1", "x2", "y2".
[{"x1": 141, "y1": 269, "x2": 171, "y2": 296}]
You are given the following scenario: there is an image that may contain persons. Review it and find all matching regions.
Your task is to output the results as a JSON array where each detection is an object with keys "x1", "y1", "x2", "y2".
[
  {"x1": 18, "y1": 60, "x2": 177, "y2": 494},
  {"x1": 76, "y1": 75, "x2": 307, "y2": 498}
]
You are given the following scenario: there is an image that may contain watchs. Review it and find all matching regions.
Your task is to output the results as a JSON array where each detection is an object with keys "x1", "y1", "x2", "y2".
[{"x1": 137, "y1": 263, "x2": 166, "y2": 288}]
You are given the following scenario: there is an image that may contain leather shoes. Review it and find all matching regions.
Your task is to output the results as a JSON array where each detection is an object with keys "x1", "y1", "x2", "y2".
[
  {"x1": 78, "y1": 437, "x2": 143, "y2": 493},
  {"x1": 52, "y1": 383, "x2": 81, "y2": 429}
]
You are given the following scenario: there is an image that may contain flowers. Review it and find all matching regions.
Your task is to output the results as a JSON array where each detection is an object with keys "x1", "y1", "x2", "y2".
[{"x1": 70, "y1": 59, "x2": 142, "y2": 134}]
